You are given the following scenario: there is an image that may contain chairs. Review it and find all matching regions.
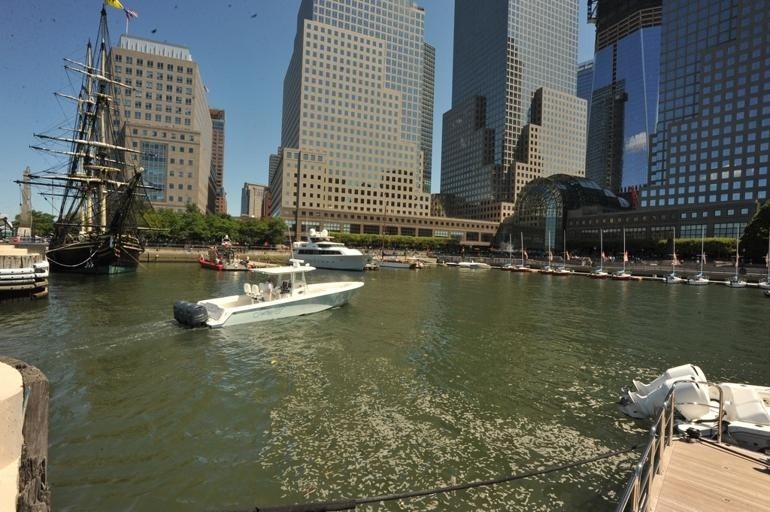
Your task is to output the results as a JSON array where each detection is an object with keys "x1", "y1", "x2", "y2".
[{"x1": 243, "y1": 282, "x2": 273, "y2": 304}]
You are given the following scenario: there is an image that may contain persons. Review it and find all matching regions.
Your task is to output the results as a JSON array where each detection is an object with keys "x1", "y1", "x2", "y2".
[
  {"x1": 243, "y1": 256, "x2": 249, "y2": 269},
  {"x1": 266, "y1": 280, "x2": 273, "y2": 301}
]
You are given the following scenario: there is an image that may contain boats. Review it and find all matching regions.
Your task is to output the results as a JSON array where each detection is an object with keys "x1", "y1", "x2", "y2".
[
  {"x1": 618, "y1": 365, "x2": 769, "y2": 450},
  {"x1": 293, "y1": 226, "x2": 375, "y2": 271},
  {"x1": 380, "y1": 256, "x2": 425, "y2": 270},
  {"x1": 200, "y1": 236, "x2": 277, "y2": 270},
  {"x1": 173, "y1": 260, "x2": 364, "y2": 329}
]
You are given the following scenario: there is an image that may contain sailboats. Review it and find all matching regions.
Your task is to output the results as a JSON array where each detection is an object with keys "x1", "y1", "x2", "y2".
[{"x1": 14, "y1": 0, "x2": 157, "y2": 273}]
